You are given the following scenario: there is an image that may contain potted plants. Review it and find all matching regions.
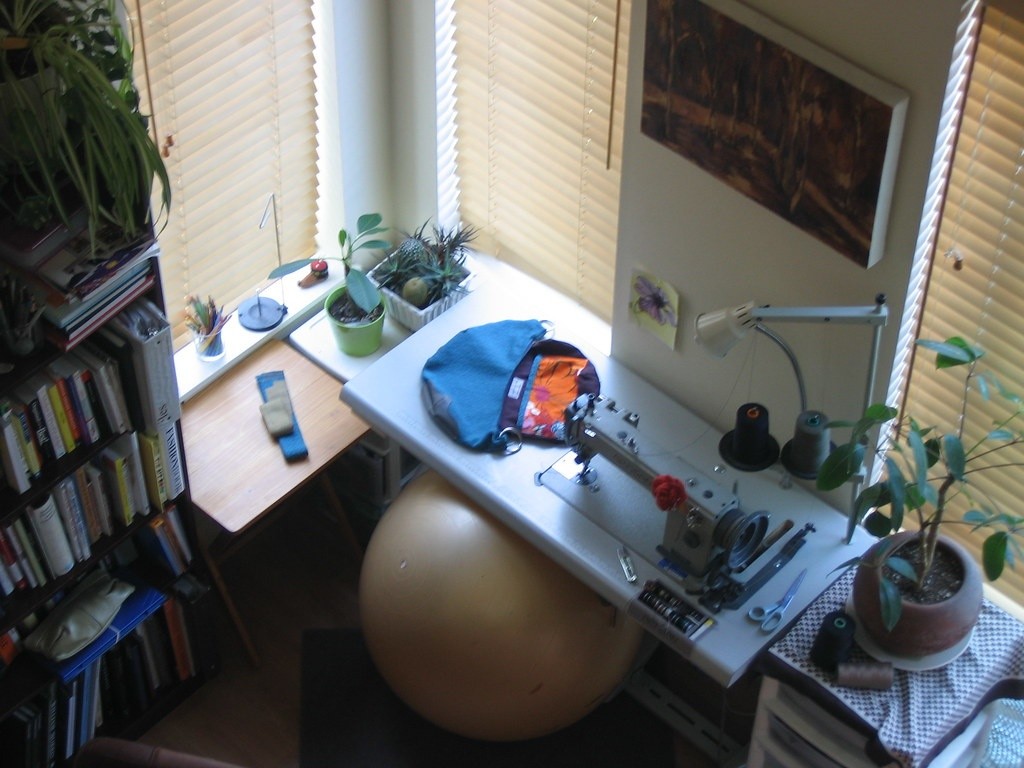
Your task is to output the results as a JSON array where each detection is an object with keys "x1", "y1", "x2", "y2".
[
  {"x1": 365, "y1": 216, "x2": 483, "y2": 331},
  {"x1": 1, "y1": 1, "x2": 171, "y2": 274},
  {"x1": 816, "y1": 333, "x2": 1024, "y2": 675},
  {"x1": 267, "y1": 214, "x2": 396, "y2": 358}
]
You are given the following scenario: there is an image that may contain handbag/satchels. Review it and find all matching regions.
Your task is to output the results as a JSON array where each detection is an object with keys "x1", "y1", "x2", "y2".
[{"x1": 420, "y1": 314, "x2": 600, "y2": 455}]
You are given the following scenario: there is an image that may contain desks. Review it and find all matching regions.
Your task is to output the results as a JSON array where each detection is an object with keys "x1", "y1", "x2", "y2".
[{"x1": 177, "y1": 339, "x2": 401, "y2": 671}]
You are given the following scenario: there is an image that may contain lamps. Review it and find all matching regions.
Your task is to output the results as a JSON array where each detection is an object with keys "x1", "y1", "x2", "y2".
[{"x1": 693, "y1": 291, "x2": 807, "y2": 489}]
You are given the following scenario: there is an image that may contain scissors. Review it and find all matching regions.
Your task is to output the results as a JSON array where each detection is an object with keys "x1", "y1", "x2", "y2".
[
  {"x1": 749, "y1": 569, "x2": 808, "y2": 633},
  {"x1": 616, "y1": 545, "x2": 638, "y2": 583}
]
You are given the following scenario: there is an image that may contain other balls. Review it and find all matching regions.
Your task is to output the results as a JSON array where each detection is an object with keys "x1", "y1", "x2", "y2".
[{"x1": 356, "y1": 467, "x2": 645, "y2": 742}]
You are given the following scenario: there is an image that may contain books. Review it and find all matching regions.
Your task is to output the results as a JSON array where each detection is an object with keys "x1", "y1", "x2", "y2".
[{"x1": 1, "y1": 178, "x2": 221, "y2": 768}]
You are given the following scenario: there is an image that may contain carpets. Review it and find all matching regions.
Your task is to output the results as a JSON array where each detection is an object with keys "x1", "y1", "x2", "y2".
[{"x1": 297, "y1": 625, "x2": 681, "y2": 767}]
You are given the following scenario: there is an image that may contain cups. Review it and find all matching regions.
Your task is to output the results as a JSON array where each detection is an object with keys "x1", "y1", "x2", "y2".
[{"x1": 192, "y1": 330, "x2": 224, "y2": 362}]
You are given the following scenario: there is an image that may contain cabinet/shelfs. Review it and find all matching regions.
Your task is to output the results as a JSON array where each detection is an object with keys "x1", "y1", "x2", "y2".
[
  {"x1": 743, "y1": 571, "x2": 1024, "y2": 768},
  {"x1": 0, "y1": 0, "x2": 223, "y2": 747}
]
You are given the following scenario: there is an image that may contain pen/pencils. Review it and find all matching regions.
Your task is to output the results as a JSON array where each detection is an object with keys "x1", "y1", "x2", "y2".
[{"x1": 183, "y1": 294, "x2": 224, "y2": 334}]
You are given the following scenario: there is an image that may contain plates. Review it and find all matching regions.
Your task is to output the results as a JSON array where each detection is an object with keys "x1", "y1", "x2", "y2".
[{"x1": 845, "y1": 589, "x2": 976, "y2": 670}]
